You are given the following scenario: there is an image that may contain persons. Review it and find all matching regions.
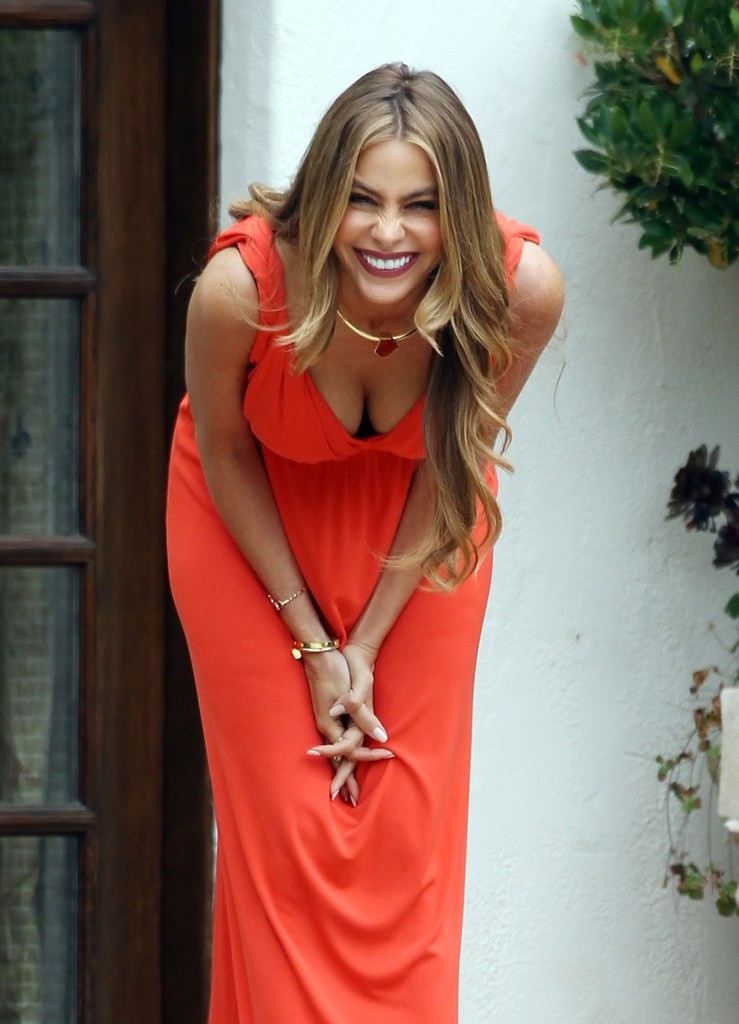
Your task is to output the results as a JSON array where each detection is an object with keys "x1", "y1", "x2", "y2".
[{"x1": 169, "y1": 60, "x2": 565, "y2": 1023}]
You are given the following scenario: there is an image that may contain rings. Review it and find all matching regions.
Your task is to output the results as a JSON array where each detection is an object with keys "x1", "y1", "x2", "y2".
[{"x1": 332, "y1": 736, "x2": 343, "y2": 761}]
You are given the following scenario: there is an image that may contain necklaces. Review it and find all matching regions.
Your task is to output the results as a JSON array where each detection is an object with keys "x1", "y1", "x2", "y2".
[{"x1": 334, "y1": 306, "x2": 420, "y2": 358}]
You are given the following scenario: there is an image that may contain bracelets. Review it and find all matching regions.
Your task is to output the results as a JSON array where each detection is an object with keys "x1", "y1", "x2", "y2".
[
  {"x1": 291, "y1": 638, "x2": 341, "y2": 660},
  {"x1": 268, "y1": 583, "x2": 308, "y2": 612}
]
